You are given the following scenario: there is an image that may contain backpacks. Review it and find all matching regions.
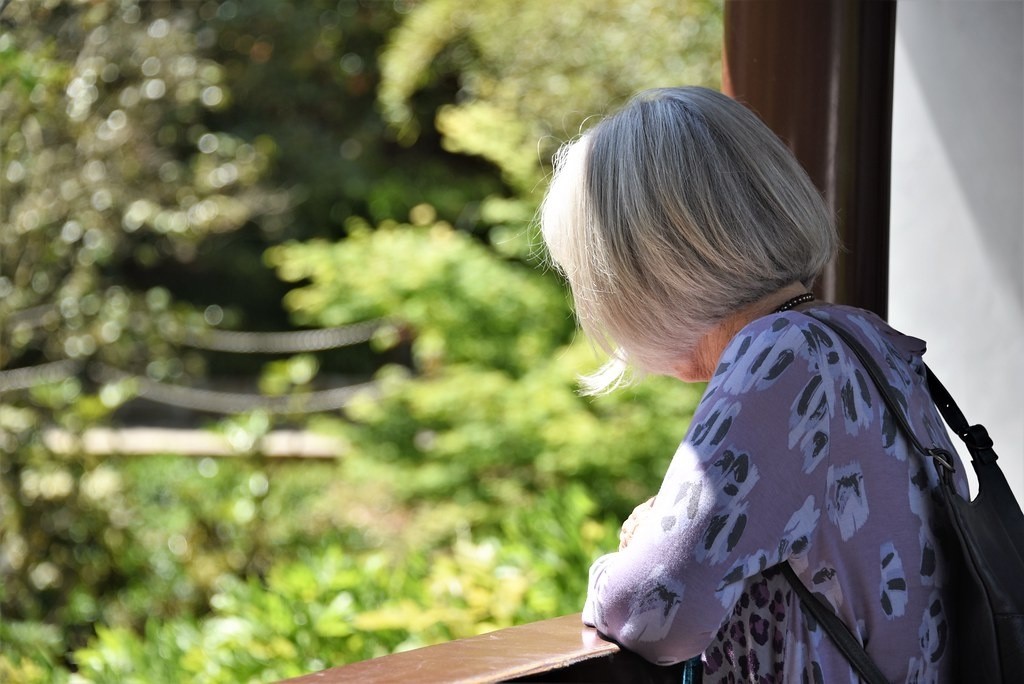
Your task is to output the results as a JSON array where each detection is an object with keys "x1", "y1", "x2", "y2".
[{"x1": 777, "y1": 311, "x2": 1024, "y2": 684}]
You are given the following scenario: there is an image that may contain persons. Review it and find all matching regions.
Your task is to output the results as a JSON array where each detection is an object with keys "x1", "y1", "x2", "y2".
[{"x1": 540, "y1": 84, "x2": 967, "y2": 684}]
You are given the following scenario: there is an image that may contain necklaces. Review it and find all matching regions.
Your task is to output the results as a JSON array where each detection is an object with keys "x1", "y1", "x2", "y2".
[{"x1": 771, "y1": 292, "x2": 813, "y2": 312}]
